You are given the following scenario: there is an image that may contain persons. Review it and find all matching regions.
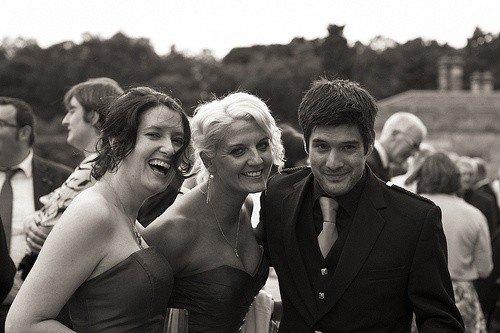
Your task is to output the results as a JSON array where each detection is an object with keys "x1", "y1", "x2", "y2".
[{"x1": 0, "y1": 77, "x2": 500, "y2": 333}]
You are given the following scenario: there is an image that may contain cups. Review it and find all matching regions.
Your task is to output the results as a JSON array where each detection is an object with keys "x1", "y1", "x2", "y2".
[{"x1": 163, "y1": 308, "x2": 188, "y2": 333}]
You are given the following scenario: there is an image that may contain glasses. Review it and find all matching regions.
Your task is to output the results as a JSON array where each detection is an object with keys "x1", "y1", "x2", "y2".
[{"x1": 0, "y1": 118, "x2": 17, "y2": 128}]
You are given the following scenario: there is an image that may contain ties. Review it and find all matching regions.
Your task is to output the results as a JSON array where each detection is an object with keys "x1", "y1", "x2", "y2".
[
  {"x1": 317, "y1": 197, "x2": 338, "y2": 259},
  {"x1": 0, "y1": 167, "x2": 22, "y2": 254}
]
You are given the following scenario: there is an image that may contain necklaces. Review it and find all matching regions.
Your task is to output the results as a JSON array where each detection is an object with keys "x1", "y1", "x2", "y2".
[
  {"x1": 207, "y1": 195, "x2": 241, "y2": 258},
  {"x1": 101, "y1": 176, "x2": 142, "y2": 248}
]
